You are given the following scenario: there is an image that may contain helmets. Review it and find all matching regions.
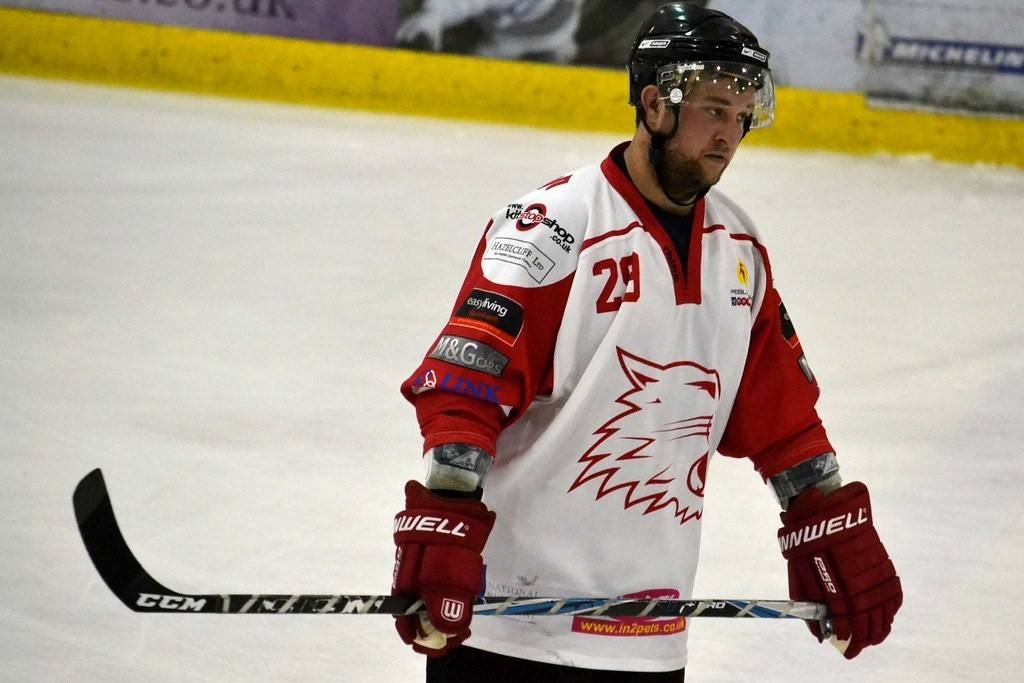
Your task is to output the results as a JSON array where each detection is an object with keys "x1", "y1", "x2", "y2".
[{"x1": 627, "y1": 2, "x2": 770, "y2": 103}]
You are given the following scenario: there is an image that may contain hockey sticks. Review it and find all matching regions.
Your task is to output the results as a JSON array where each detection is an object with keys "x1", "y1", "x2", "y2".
[{"x1": 70, "y1": 466, "x2": 832, "y2": 623}]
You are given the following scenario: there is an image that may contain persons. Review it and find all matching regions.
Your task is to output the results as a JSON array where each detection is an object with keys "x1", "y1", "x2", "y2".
[
  {"x1": 388, "y1": 0, "x2": 904, "y2": 681},
  {"x1": 392, "y1": 0, "x2": 588, "y2": 67}
]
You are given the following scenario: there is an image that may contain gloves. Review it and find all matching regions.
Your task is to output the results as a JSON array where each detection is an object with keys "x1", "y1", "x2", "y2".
[
  {"x1": 778, "y1": 480, "x2": 903, "y2": 659},
  {"x1": 390, "y1": 479, "x2": 497, "y2": 658}
]
